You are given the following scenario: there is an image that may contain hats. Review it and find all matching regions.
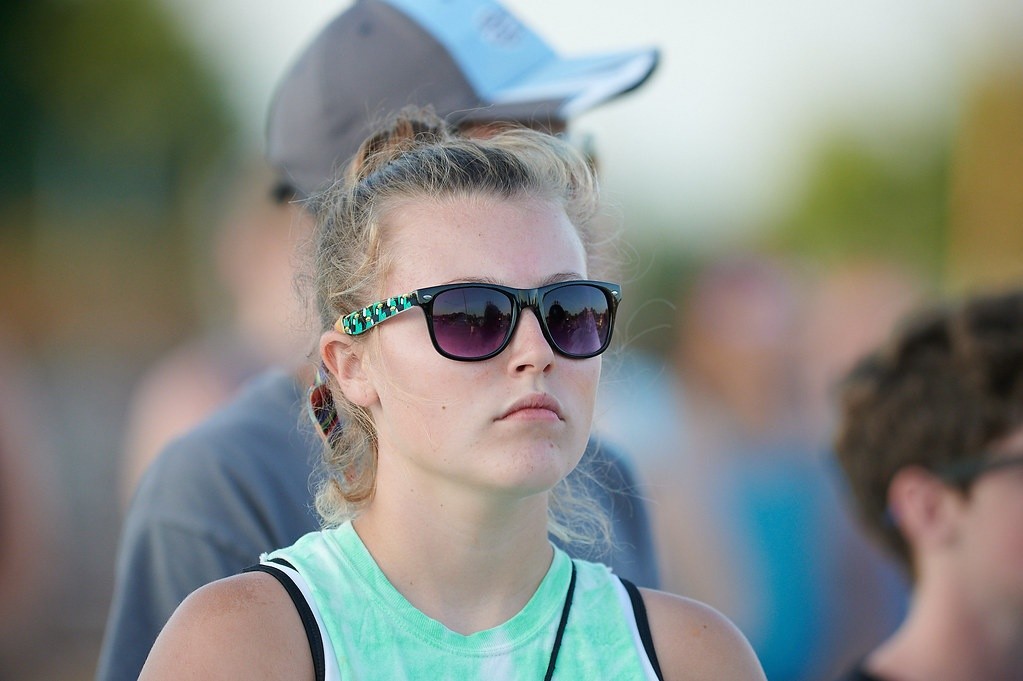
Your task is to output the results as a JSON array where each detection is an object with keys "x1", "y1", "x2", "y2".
[{"x1": 264, "y1": 0, "x2": 658, "y2": 215}]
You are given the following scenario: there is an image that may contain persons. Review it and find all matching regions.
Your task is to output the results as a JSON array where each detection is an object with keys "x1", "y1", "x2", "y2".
[
  {"x1": 133, "y1": 190, "x2": 737, "y2": 612},
  {"x1": 835, "y1": 291, "x2": 1022, "y2": 681},
  {"x1": 137, "y1": 120, "x2": 766, "y2": 681},
  {"x1": 436, "y1": 304, "x2": 508, "y2": 355},
  {"x1": 548, "y1": 305, "x2": 608, "y2": 353},
  {"x1": 97, "y1": 0, "x2": 661, "y2": 680}
]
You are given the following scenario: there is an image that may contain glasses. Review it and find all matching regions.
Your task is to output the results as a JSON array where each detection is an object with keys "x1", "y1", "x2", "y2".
[{"x1": 315, "y1": 281, "x2": 622, "y2": 386}]
